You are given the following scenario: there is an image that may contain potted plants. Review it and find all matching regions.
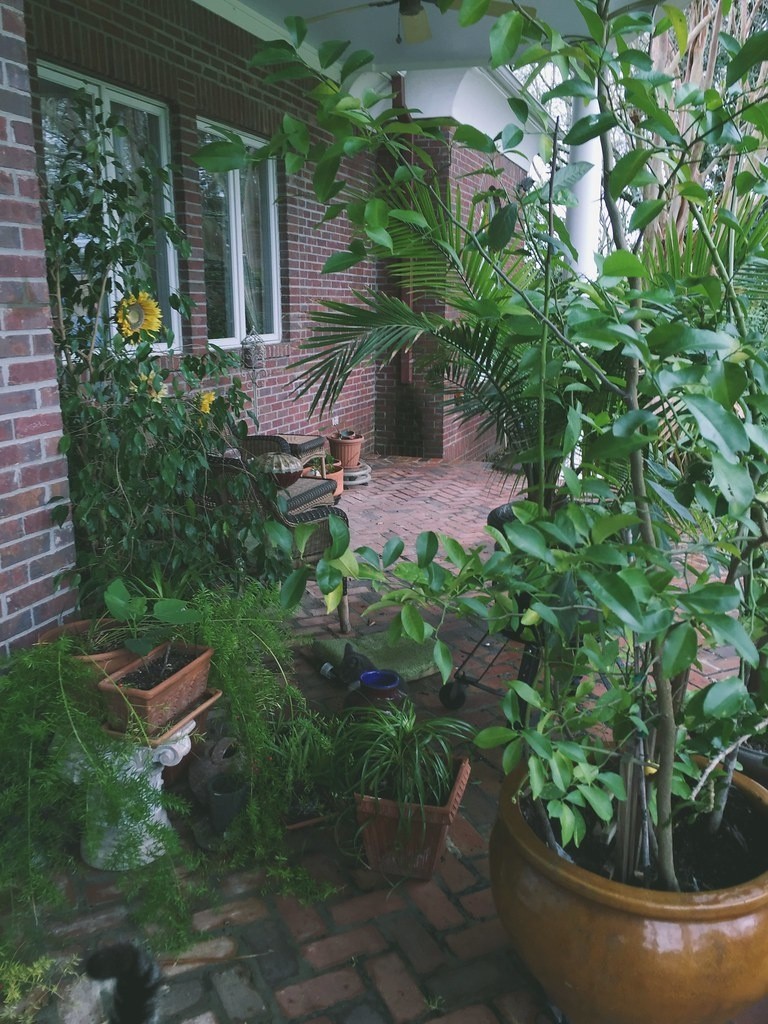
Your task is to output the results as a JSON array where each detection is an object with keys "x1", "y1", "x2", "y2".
[
  {"x1": 300, "y1": 453, "x2": 344, "y2": 505},
  {"x1": 0, "y1": 573, "x2": 481, "y2": 1024},
  {"x1": 317, "y1": 425, "x2": 364, "y2": 469},
  {"x1": 184, "y1": 1, "x2": 768, "y2": 1024}
]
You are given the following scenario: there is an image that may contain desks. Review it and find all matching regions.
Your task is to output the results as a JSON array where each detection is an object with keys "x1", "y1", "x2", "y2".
[
  {"x1": 271, "y1": 433, "x2": 326, "y2": 480},
  {"x1": 276, "y1": 477, "x2": 337, "y2": 516}
]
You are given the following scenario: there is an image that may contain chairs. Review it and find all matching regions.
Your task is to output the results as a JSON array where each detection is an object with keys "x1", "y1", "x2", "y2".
[
  {"x1": 182, "y1": 455, "x2": 352, "y2": 634},
  {"x1": 238, "y1": 436, "x2": 292, "y2": 458}
]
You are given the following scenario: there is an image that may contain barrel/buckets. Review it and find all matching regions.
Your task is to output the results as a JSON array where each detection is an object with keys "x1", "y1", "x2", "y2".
[{"x1": 360, "y1": 669, "x2": 400, "y2": 705}]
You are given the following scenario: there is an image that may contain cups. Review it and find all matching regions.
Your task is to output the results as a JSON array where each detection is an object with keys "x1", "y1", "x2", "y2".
[{"x1": 207, "y1": 774, "x2": 245, "y2": 832}]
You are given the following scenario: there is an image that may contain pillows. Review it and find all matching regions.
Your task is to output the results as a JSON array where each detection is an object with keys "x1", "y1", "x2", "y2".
[{"x1": 256, "y1": 451, "x2": 304, "y2": 489}]
